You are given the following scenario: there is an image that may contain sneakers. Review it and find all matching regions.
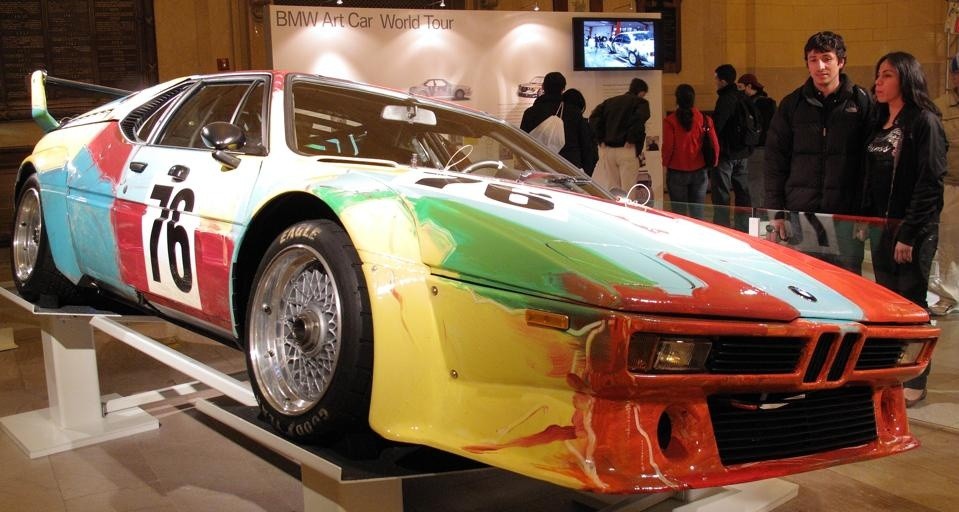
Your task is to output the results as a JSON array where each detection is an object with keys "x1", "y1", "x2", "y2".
[{"x1": 903, "y1": 387, "x2": 928, "y2": 410}]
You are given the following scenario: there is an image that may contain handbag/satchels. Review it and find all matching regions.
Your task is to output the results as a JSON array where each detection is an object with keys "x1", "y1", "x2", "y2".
[
  {"x1": 527, "y1": 101, "x2": 568, "y2": 156},
  {"x1": 701, "y1": 130, "x2": 717, "y2": 168}
]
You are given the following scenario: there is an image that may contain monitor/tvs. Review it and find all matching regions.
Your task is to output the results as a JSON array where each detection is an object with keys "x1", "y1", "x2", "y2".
[{"x1": 572, "y1": 16, "x2": 664, "y2": 71}]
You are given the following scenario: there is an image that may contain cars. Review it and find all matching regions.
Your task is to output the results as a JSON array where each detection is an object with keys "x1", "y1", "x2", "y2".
[
  {"x1": 9, "y1": 65, "x2": 942, "y2": 496},
  {"x1": 594, "y1": 31, "x2": 655, "y2": 66}
]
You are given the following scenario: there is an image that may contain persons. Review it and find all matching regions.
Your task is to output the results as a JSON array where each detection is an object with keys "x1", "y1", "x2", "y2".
[
  {"x1": 870, "y1": 84, "x2": 889, "y2": 129},
  {"x1": 737, "y1": 73, "x2": 776, "y2": 222},
  {"x1": 554, "y1": 88, "x2": 599, "y2": 177},
  {"x1": 584, "y1": 34, "x2": 614, "y2": 49},
  {"x1": 649, "y1": 140, "x2": 659, "y2": 151},
  {"x1": 764, "y1": 31, "x2": 878, "y2": 277},
  {"x1": 590, "y1": 78, "x2": 651, "y2": 200},
  {"x1": 521, "y1": 72, "x2": 567, "y2": 133},
  {"x1": 862, "y1": 50, "x2": 948, "y2": 407},
  {"x1": 712, "y1": 64, "x2": 751, "y2": 232},
  {"x1": 661, "y1": 83, "x2": 721, "y2": 219}
]
